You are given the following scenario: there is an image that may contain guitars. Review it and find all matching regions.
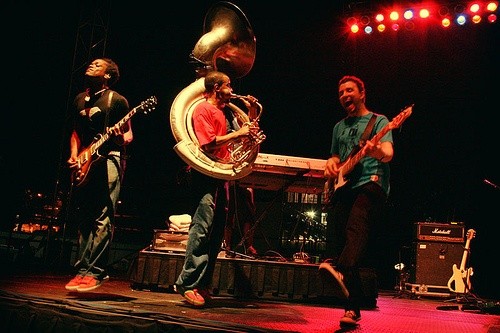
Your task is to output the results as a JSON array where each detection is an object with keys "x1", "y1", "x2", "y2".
[
  {"x1": 67, "y1": 95, "x2": 158, "y2": 191},
  {"x1": 318, "y1": 103, "x2": 416, "y2": 208},
  {"x1": 446, "y1": 228, "x2": 476, "y2": 295}
]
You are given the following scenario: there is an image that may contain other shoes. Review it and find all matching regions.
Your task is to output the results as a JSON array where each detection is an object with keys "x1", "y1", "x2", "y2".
[{"x1": 173, "y1": 283, "x2": 205, "y2": 306}]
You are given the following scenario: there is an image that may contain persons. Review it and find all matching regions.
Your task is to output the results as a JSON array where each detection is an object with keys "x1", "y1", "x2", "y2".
[
  {"x1": 316, "y1": 76, "x2": 394, "y2": 328},
  {"x1": 173, "y1": 71, "x2": 257, "y2": 305},
  {"x1": 65, "y1": 58, "x2": 133, "y2": 291}
]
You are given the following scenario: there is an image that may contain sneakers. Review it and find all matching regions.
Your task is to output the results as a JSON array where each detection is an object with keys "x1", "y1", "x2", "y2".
[
  {"x1": 77, "y1": 271, "x2": 109, "y2": 291},
  {"x1": 339, "y1": 309, "x2": 362, "y2": 325},
  {"x1": 65, "y1": 269, "x2": 88, "y2": 290},
  {"x1": 319, "y1": 263, "x2": 349, "y2": 299}
]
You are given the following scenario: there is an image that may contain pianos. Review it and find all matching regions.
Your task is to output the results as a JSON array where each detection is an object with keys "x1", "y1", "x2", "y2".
[{"x1": 234, "y1": 151, "x2": 338, "y2": 192}]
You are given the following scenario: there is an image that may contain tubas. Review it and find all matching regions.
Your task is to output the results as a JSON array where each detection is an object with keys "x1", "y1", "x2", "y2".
[{"x1": 168, "y1": 1, "x2": 265, "y2": 181}]
